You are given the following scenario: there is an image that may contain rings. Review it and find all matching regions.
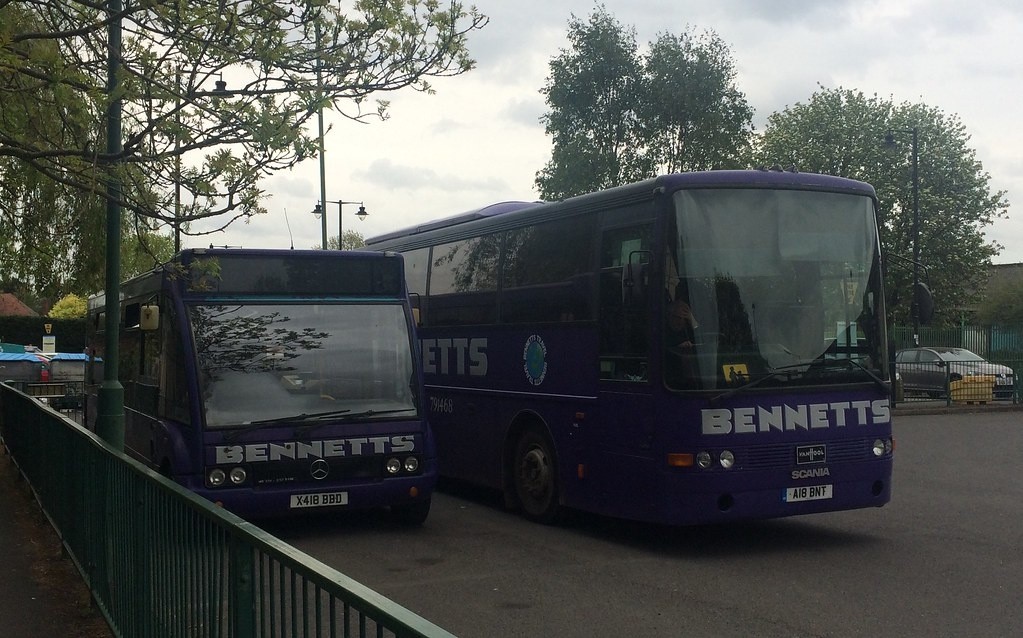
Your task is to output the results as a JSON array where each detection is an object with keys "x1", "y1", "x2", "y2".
[{"x1": 686, "y1": 344, "x2": 689, "y2": 346}]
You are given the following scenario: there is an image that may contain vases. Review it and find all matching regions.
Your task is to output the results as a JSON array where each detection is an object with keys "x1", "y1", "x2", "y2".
[{"x1": 41, "y1": 370, "x2": 48, "y2": 381}]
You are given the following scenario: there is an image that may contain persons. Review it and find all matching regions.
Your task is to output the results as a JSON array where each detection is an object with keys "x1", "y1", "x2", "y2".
[{"x1": 663, "y1": 298, "x2": 707, "y2": 354}]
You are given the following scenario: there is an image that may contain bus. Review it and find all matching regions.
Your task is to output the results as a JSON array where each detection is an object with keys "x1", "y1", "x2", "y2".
[
  {"x1": 352, "y1": 168, "x2": 896, "y2": 529},
  {"x1": 82, "y1": 246, "x2": 432, "y2": 542}
]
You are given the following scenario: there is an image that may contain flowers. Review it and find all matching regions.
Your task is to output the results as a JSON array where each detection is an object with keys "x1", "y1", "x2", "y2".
[{"x1": 39, "y1": 359, "x2": 50, "y2": 370}]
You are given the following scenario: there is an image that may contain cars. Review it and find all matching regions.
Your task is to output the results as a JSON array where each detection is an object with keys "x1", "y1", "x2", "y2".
[{"x1": 895, "y1": 347, "x2": 1016, "y2": 399}]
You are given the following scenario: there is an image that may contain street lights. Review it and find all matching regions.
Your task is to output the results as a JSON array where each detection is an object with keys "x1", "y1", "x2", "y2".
[
  {"x1": 311, "y1": 200, "x2": 370, "y2": 251},
  {"x1": 174, "y1": 65, "x2": 234, "y2": 256},
  {"x1": 882, "y1": 127, "x2": 922, "y2": 348}
]
